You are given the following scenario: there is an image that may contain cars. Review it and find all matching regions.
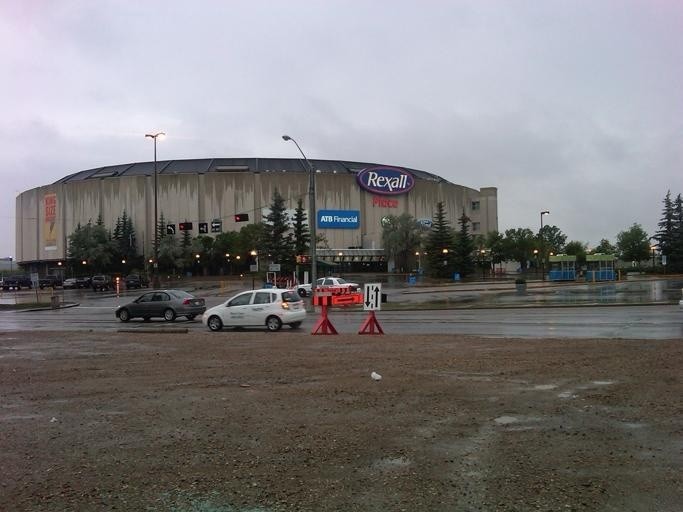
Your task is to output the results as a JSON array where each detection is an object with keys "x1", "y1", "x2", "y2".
[
  {"x1": 62, "y1": 278, "x2": 83, "y2": 287},
  {"x1": 115, "y1": 290, "x2": 206, "y2": 321},
  {"x1": 295, "y1": 276, "x2": 360, "y2": 297},
  {"x1": 202, "y1": 289, "x2": 306, "y2": 331}
]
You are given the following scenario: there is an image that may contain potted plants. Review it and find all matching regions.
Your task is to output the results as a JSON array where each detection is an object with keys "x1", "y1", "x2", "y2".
[{"x1": 515, "y1": 278, "x2": 527, "y2": 292}]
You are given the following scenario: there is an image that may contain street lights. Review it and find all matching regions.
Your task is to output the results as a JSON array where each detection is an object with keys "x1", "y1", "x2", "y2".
[
  {"x1": 8, "y1": 257, "x2": 12, "y2": 271},
  {"x1": 145, "y1": 131, "x2": 166, "y2": 290},
  {"x1": 282, "y1": 135, "x2": 317, "y2": 312},
  {"x1": 533, "y1": 250, "x2": 539, "y2": 278},
  {"x1": 226, "y1": 253, "x2": 240, "y2": 275},
  {"x1": 481, "y1": 250, "x2": 486, "y2": 280},
  {"x1": 442, "y1": 249, "x2": 449, "y2": 278},
  {"x1": 540, "y1": 211, "x2": 550, "y2": 280},
  {"x1": 415, "y1": 250, "x2": 428, "y2": 268}
]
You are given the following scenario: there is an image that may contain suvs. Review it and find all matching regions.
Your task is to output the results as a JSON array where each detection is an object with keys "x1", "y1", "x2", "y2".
[
  {"x1": 0, "y1": 275, "x2": 33, "y2": 291},
  {"x1": 92, "y1": 275, "x2": 110, "y2": 292},
  {"x1": 124, "y1": 275, "x2": 149, "y2": 288},
  {"x1": 39, "y1": 275, "x2": 62, "y2": 290}
]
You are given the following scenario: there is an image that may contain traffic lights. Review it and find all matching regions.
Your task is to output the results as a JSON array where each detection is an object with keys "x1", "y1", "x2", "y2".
[
  {"x1": 178, "y1": 222, "x2": 192, "y2": 230},
  {"x1": 235, "y1": 214, "x2": 249, "y2": 222}
]
